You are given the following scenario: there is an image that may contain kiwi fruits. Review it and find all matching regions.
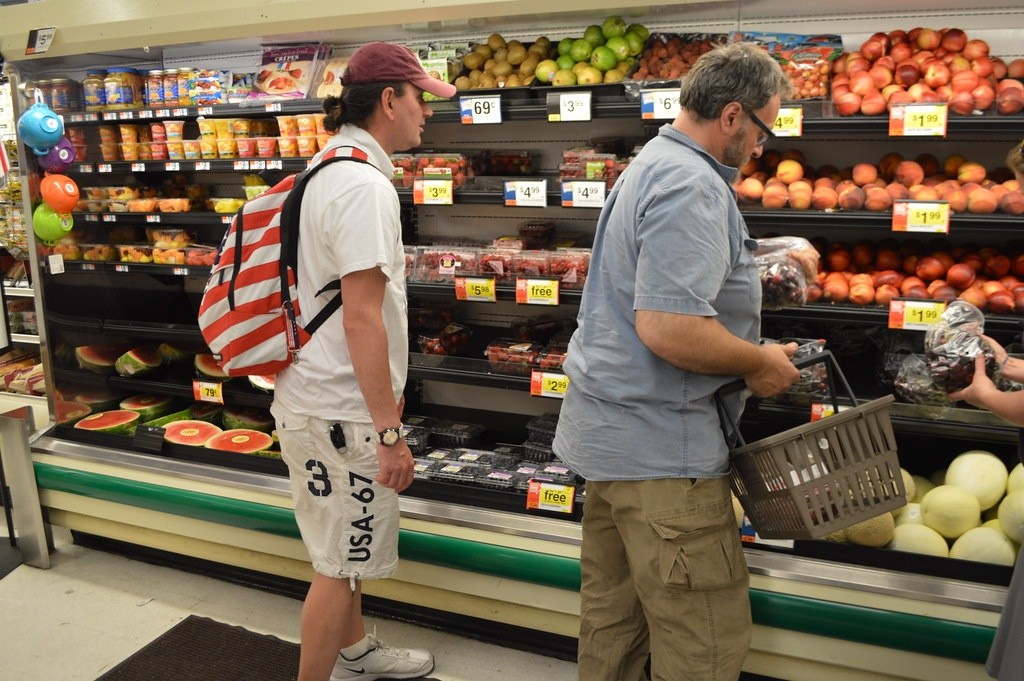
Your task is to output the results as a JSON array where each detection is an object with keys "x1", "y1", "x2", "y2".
[{"x1": 452, "y1": 31, "x2": 551, "y2": 88}]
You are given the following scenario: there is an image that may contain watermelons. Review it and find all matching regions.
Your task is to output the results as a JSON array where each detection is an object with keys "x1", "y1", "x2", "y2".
[
  {"x1": 70, "y1": 338, "x2": 286, "y2": 462},
  {"x1": 52, "y1": 380, "x2": 129, "y2": 430}
]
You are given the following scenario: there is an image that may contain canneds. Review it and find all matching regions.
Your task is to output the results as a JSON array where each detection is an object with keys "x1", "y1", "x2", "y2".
[
  {"x1": 32, "y1": 63, "x2": 148, "y2": 114},
  {"x1": 147, "y1": 67, "x2": 196, "y2": 109}
]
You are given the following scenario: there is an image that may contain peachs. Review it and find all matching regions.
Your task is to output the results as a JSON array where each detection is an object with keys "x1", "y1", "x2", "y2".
[
  {"x1": 731, "y1": 150, "x2": 1023, "y2": 221},
  {"x1": 806, "y1": 241, "x2": 1024, "y2": 317},
  {"x1": 828, "y1": 27, "x2": 1024, "y2": 115}
]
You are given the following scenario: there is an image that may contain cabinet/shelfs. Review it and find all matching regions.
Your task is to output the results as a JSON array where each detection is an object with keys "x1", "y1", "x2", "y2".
[{"x1": 0, "y1": 0, "x2": 1024, "y2": 681}]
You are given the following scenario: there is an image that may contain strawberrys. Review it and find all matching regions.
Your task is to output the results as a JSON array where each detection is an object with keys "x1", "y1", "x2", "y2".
[
  {"x1": 409, "y1": 299, "x2": 471, "y2": 355},
  {"x1": 487, "y1": 147, "x2": 534, "y2": 176},
  {"x1": 486, "y1": 316, "x2": 566, "y2": 376},
  {"x1": 390, "y1": 151, "x2": 483, "y2": 187}
]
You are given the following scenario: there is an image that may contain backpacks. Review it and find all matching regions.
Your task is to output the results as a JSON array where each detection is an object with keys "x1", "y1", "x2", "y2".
[{"x1": 196, "y1": 145, "x2": 388, "y2": 380}]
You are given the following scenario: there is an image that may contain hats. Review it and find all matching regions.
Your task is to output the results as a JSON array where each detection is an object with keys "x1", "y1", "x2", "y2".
[{"x1": 339, "y1": 40, "x2": 457, "y2": 99}]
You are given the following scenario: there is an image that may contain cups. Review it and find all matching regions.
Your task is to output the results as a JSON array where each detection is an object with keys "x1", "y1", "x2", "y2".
[{"x1": 63, "y1": 103, "x2": 339, "y2": 163}]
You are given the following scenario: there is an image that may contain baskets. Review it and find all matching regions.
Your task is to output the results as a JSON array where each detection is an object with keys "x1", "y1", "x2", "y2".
[{"x1": 719, "y1": 348, "x2": 908, "y2": 542}]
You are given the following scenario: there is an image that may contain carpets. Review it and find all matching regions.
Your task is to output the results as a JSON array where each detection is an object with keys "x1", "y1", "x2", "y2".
[{"x1": 93, "y1": 612, "x2": 444, "y2": 681}]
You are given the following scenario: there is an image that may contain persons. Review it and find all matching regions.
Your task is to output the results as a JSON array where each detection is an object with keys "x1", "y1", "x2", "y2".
[
  {"x1": 271, "y1": 42, "x2": 458, "y2": 680},
  {"x1": 552, "y1": 38, "x2": 819, "y2": 681},
  {"x1": 952, "y1": 334, "x2": 1024, "y2": 681}
]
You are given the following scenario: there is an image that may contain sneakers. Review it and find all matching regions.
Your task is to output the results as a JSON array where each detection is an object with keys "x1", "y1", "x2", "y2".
[{"x1": 329, "y1": 625, "x2": 435, "y2": 681}]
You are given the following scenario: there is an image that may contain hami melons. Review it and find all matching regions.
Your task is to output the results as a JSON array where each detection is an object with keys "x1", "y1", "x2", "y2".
[{"x1": 815, "y1": 451, "x2": 1024, "y2": 567}]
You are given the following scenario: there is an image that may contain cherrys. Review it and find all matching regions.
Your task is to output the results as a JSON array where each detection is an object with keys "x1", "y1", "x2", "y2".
[
  {"x1": 751, "y1": 258, "x2": 804, "y2": 314},
  {"x1": 760, "y1": 325, "x2": 1023, "y2": 407}
]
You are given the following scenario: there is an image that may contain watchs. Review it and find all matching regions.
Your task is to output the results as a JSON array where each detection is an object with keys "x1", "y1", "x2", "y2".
[{"x1": 373, "y1": 422, "x2": 404, "y2": 447}]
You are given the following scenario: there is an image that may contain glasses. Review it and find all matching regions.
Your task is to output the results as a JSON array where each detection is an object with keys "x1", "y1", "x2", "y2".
[{"x1": 744, "y1": 108, "x2": 776, "y2": 148}]
